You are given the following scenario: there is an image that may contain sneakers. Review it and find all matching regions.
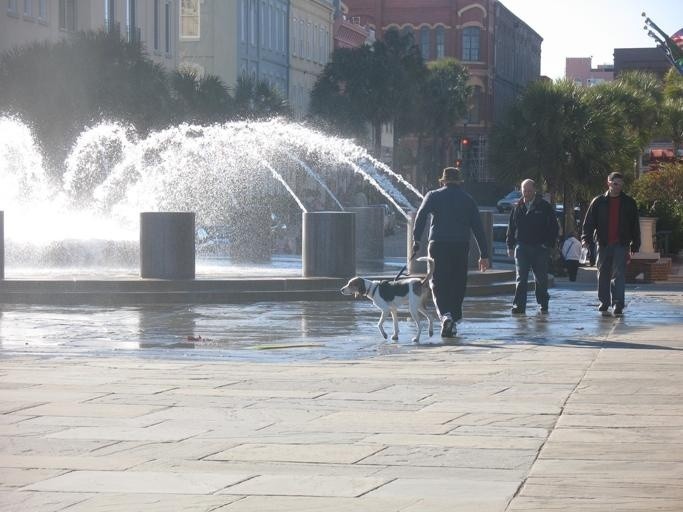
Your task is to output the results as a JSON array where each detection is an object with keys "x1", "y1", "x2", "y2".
[
  {"x1": 512, "y1": 306, "x2": 523, "y2": 314},
  {"x1": 613, "y1": 303, "x2": 623, "y2": 314},
  {"x1": 598, "y1": 304, "x2": 609, "y2": 311},
  {"x1": 441, "y1": 311, "x2": 457, "y2": 337},
  {"x1": 540, "y1": 300, "x2": 548, "y2": 311}
]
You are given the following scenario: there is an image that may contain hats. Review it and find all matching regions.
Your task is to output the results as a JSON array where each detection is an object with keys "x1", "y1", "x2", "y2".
[{"x1": 438, "y1": 166, "x2": 465, "y2": 184}]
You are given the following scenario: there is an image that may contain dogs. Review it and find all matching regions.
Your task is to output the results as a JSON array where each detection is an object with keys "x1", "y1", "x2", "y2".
[{"x1": 339, "y1": 256, "x2": 436, "y2": 342}]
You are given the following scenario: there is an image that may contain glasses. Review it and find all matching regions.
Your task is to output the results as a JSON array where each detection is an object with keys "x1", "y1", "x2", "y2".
[{"x1": 609, "y1": 180, "x2": 624, "y2": 188}]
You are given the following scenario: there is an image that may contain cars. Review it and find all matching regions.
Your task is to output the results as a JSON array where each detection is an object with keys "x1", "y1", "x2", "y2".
[{"x1": 496, "y1": 190, "x2": 523, "y2": 213}]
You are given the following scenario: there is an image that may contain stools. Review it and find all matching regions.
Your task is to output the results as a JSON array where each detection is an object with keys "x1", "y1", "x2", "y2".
[{"x1": 575, "y1": 265, "x2": 598, "y2": 284}]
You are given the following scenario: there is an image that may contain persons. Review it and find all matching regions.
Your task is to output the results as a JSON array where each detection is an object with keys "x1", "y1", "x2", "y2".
[
  {"x1": 562, "y1": 231, "x2": 582, "y2": 281},
  {"x1": 412, "y1": 165, "x2": 489, "y2": 336},
  {"x1": 582, "y1": 171, "x2": 643, "y2": 315},
  {"x1": 505, "y1": 179, "x2": 560, "y2": 315}
]
